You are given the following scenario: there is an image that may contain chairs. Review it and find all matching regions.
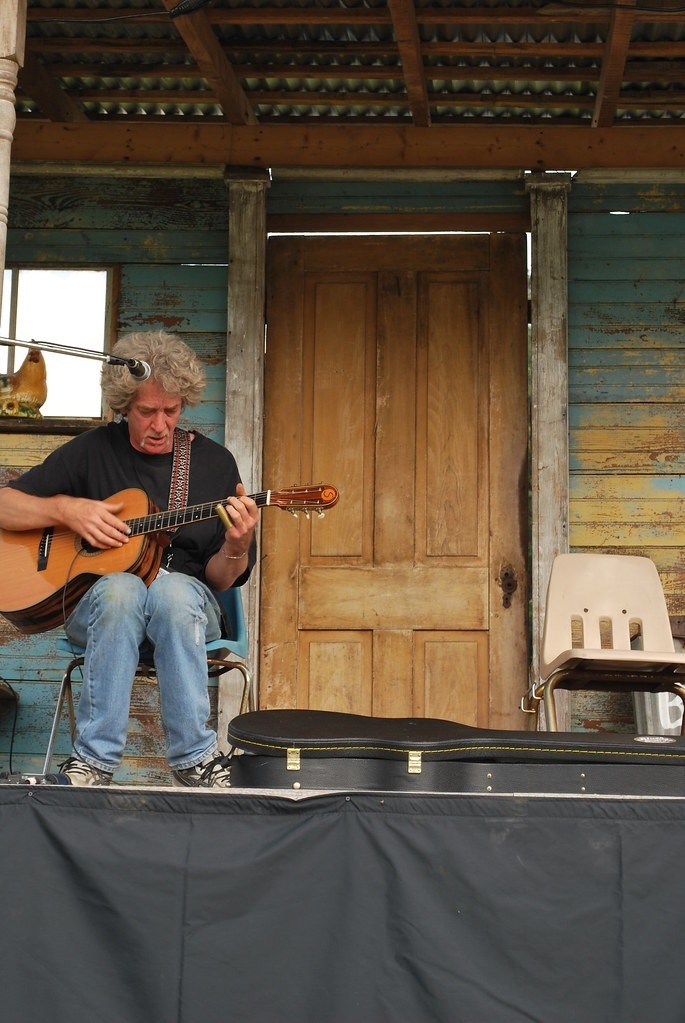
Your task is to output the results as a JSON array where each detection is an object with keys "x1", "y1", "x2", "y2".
[
  {"x1": 518, "y1": 553, "x2": 685, "y2": 737},
  {"x1": 40, "y1": 584, "x2": 255, "y2": 781}
]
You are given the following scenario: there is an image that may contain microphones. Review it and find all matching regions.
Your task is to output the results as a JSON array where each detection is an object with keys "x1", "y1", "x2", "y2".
[{"x1": 128, "y1": 359, "x2": 152, "y2": 382}]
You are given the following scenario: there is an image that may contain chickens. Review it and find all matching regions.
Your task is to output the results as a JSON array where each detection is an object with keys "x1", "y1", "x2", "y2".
[{"x1": 0, "y1": 339, "x2": 48, "y2": 406}]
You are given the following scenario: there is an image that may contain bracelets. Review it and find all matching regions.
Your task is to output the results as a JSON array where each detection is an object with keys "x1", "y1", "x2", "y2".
[{"x1": 222, "y1": 542, "x2": 249, "y2": 560}]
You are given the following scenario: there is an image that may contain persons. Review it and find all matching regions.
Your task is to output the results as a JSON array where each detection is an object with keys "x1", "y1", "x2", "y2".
[{"x1": 0, "y1": 330, "x2": 260, "y2": 786}]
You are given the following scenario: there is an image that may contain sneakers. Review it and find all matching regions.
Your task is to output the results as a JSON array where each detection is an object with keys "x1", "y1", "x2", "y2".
[
  {"x1": 173, "y1": 745, "x2": 233, "y2": 788},
  {"x1": 45, "y1": 756, "x2": 114, "y2": 786}
]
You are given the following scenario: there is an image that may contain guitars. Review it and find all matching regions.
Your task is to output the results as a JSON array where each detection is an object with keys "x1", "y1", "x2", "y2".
[{"x1": 0, "y1": 480, "x2": 340, "y2": 635}]
